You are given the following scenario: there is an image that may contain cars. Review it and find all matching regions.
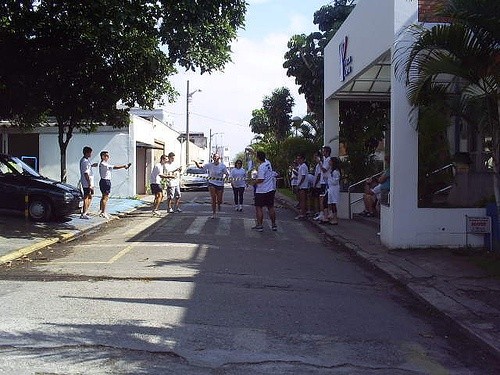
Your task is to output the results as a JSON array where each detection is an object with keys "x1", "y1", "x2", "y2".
[
  {"x1": 179, "y1": 166, "x2": 209, "y2": 190},
  {"x1": 0, "y1": 153, "x2": 84, "y2": 224}
]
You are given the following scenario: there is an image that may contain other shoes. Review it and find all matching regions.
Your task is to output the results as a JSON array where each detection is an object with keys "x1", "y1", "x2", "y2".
[
  {"x1": 293, "y1": 211, "x2": 338, "y2": 225},
  {"x1": 251, "y1": 225, "x2": 264, "y2": 232},
  {"x1": 235, "y1": 205, "x2": 243, "y2": 212},
  {"x1": 272, "y1": 224, "x2": 278, "y2": 231},
  {"x1": 168, "y1": 207, "x2": 174, "y2": 213},
  {"x1": 80, "y1": 214, "x2": 91, "y2": 219},
  {"x1": 176, "y1": 207, "x2": 182, "y2": 212}
]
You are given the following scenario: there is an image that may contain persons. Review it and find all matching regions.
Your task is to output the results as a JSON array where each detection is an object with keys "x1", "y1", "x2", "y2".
[
  {"x1": 363, "y1": 156, "x2": 390, "y2": 235},
  {"x1": 164, "y1": 152, "x2": 183, "y2": 212},
  {"x1": 252, "y1": 151, "x2": 279, "y2": 231},
  {"x1": 229, "y1": 160, "x2": 248, "y2": 211},
  {"x1": 151, "y1": 155, "x2": 175, "y2": 215},
  {"x1": 99, "y1": 151, "x2": 128, "y2": 219},
  {"x1": 195, "y1": 154, "x2": 229, "y2": 218},
  {"x1": 80, "y1": 147, "x2": 98, "y2": 219},
  {"x1": 290, "y1": 147, "x2": 341, "y2": 224}
]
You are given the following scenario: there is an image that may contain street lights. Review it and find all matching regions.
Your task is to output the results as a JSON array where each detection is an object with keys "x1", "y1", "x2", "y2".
[
  {"x1": 185, "y1": 89, "x2": 203, "y2": 168},
  {"x1": 209, "y1": 133, "x2": 219, "y2": 162}
]
